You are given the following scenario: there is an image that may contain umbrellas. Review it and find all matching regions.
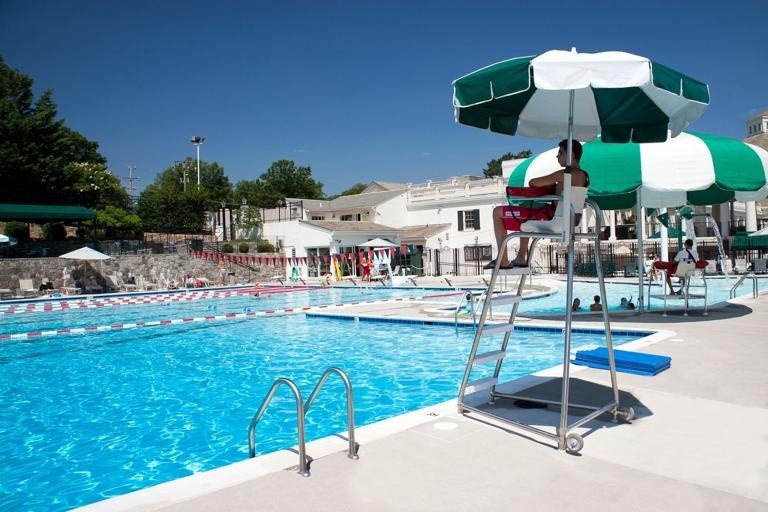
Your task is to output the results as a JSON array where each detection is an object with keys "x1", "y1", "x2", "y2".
[
  {"x1": 451, "y1": 46, "x2": 710, "y2": 244},
  {"x1": 732, "y1": 225, "x2": 768, "y2": 249},
  {"x1": 58, "y1": 246, "x2": 111, "y2": 281},
  {"x1": 0, "y1": 234, "x2": 18, "y2": 248},
  {"x1": 356, "y1": 238, "x2": 398, "y2": 248},
  {"x1": 508, "y1": 131, "x2": 768, "y2": 313}
]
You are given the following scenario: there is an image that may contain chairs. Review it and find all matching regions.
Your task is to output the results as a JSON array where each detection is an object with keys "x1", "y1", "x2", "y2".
[
  {"x1": 0, "y1": 272, "x2": 211, "y2": 300},
  {"x1": 640, "y1": 257, "x2": 768, "y2": 277},
  {"x1": 272, "y1": 267, "x2": 519, "y2": 287}
]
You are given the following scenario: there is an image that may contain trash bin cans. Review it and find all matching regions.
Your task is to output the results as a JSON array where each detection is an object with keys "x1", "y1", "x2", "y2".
[{"x1": 755, "y1": 259, "x2": 766, "y2": 274}]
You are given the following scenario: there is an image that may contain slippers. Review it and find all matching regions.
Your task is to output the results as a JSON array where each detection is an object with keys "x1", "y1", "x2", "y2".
[
  {"x1": 484, "y1": 260, "x2": 513, "y2": 269},
  {"x1": 511, "y1": 259, "x2": 529, "y2": 267}
]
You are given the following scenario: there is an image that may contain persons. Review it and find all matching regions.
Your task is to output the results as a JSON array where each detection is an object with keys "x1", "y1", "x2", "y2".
[
  {"x1": 483, "y1": 138, "x2": 589, "y2": 269},
  {"x1": 318, "y1": 272, "x2": 333, "y2": 288},
  {"x1": 361, "y1": 251, "x2": 371, "y2": 282},
  {"x1": 667, "y1": 239, "x2": 699, "y2": 294},
  {"x1": 571, "y1": 297, "x2": 636, "y2": 312}
]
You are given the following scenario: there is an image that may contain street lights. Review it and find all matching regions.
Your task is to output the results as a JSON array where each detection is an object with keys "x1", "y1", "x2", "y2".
[
  {"x1": 219, "y1": 199, "x2": 227, "y2": 242},
  {"x1": 240, "y1": 197, "x2": 247, "y2": 206},
  {"x1": 277, "y1": 200, "x2": 282, "y2": 222},
  {"x1": 188, "y1": 134, "x2": 206, "y2": 191}
]
